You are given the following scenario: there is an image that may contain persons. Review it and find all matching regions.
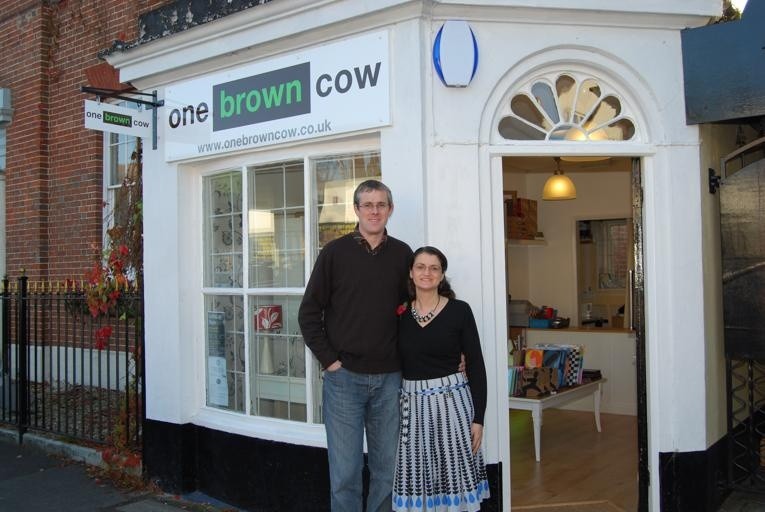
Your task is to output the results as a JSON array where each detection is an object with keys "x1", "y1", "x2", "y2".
[
  {"x1": 298, "y1": 180, "x2": 414, "y2": 512},
  {"x1": 390, "y1": 246, "x2": 491, "y2": 512}
]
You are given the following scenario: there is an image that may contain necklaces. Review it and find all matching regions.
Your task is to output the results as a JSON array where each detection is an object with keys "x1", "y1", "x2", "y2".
[{"x1": 412, "y1": 295, "x2": 441, "y2": 321}]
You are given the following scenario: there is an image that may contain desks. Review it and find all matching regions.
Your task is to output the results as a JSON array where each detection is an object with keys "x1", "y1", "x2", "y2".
[{"x1": 509, "y1": 372, "x2": 607, "y2": 463}]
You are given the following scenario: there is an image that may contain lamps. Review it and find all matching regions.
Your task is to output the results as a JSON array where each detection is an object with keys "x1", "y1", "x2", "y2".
[
  {"x1": 561, "y1": 109, "x2": 614, "y2": 162},
  {"x1": 543, "y1": 158, "x2": 578, "y2": 202},
  {"x1": 254, "y1": 304, "x2": 283, "y2": 374}
]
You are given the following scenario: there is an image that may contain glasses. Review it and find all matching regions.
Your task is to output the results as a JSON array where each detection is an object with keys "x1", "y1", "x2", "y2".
[{"x1": 358, "y1": 202, "x2": 389, "y2": 212}]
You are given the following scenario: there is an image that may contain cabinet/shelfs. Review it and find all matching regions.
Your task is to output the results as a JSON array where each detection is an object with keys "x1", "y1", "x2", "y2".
[{"x1": 509, "y1": 333, "x2": 639, "y2": 417}]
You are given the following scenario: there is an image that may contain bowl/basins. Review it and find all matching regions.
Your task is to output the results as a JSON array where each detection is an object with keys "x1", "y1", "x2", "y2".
[{"x1": 550, "y1": 321, "x2": 563, "y2": 329}]
